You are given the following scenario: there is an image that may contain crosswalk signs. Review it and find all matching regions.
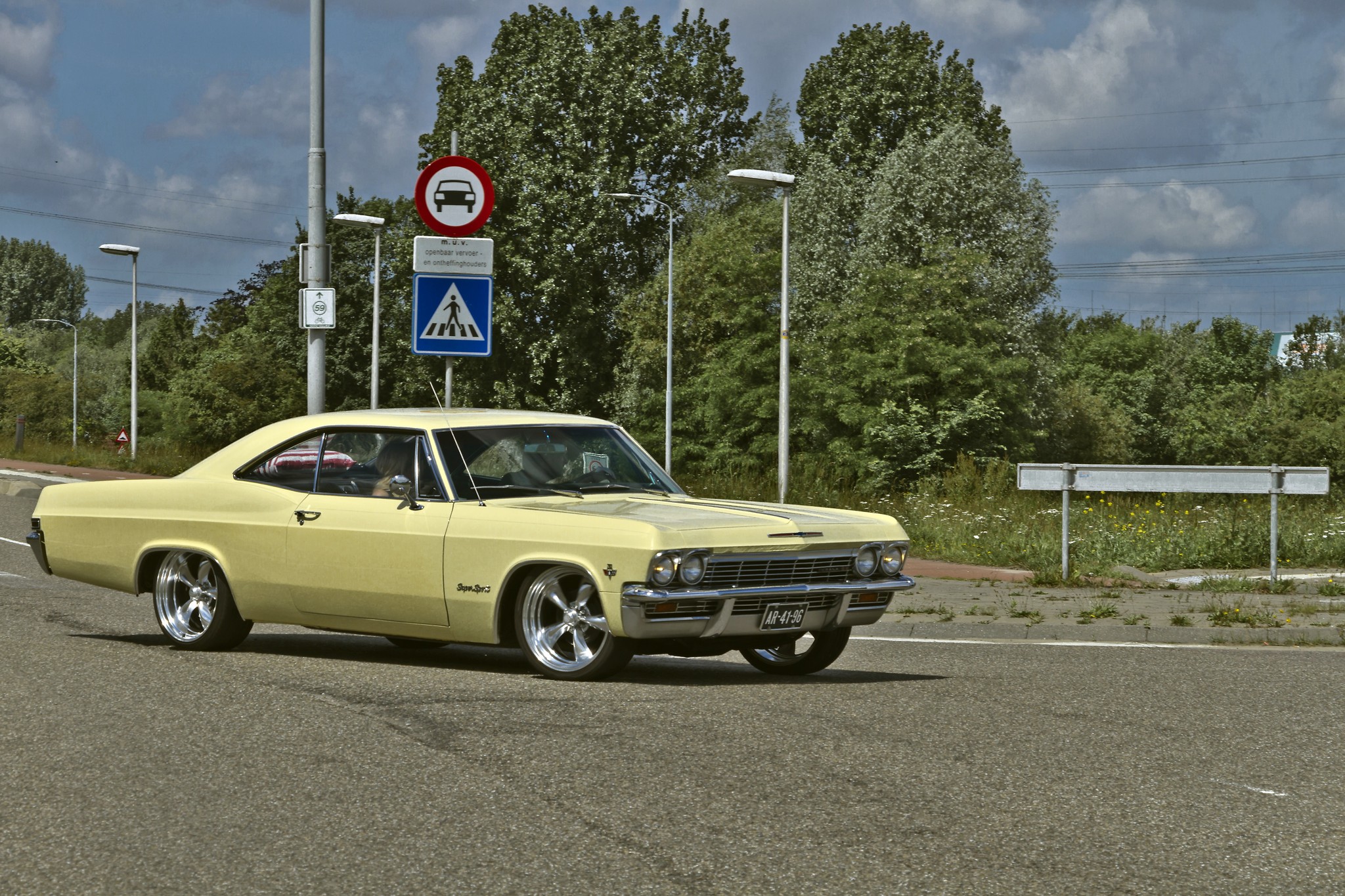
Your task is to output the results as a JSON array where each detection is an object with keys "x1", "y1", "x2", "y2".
[{"x1": 412, "y1": 276, "x2": 493, "y2": 361}]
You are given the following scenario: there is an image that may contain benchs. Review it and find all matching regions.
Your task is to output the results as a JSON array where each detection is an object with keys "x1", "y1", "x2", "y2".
[{"x1": 270, "y1": 473, "x2": 381, "y2": 494}]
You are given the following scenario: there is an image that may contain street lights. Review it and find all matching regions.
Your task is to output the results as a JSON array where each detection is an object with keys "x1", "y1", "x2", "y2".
[
  {"x1": 31, "y1": 317, "x2": 84, "y2": 450},
  {"x1": 609, "y1": 190, "x2": 678, "y2": 481},
  {"x1": 722, "y1": 167, "x2": 801, "y2": 505},
  {"x1": 99, "y1": 240, "x2": 141, "y2": 466},
  {"x1": 332, "y1": 212, "x2": 383, "y2": 404}
]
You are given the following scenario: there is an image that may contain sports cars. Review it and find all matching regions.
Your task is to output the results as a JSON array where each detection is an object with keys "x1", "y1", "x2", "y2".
[{"x1": 28, "y1": 406, "x2": 917, "y2": 679}]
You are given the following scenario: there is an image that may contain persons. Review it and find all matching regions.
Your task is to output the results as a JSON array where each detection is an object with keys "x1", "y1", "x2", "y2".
[
  {"x1": 372, "y1": 439, "x2": 436, "y2": 499},
  {"x1": 468, "y1": 429, "x2": 615, "y2": 498}
]
